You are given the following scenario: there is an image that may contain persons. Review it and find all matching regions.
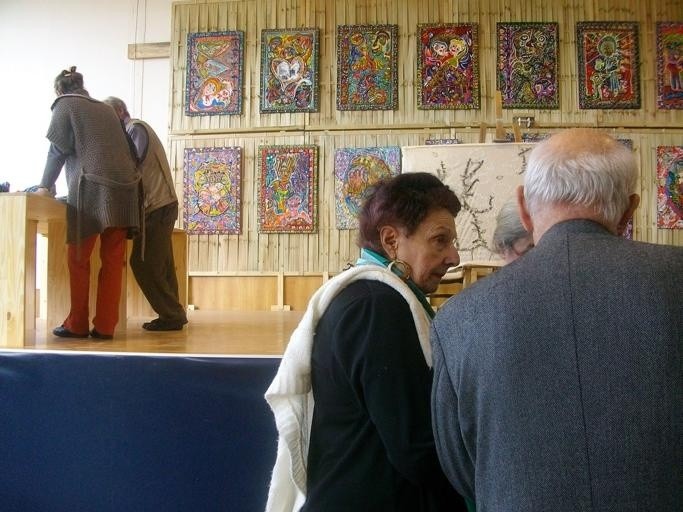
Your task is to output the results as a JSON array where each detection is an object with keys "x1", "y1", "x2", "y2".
[
  {"x1": 31, "y1": 65, "x2": 143, "y2": 340},
  {"x1": 431, "y1": 127, "x2": 682, "y2": 512},
  {"x1": 103, "y1": 93, "x2": 189, "y2": 331},
  {"x1": 283, "y1": 171, "x2": 465, "y2": 512},
  {"x1": 492, "y1": 198, "x2": 536, "y2": 275}
]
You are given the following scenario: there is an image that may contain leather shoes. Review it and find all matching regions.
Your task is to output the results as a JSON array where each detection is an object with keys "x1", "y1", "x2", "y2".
[
  {"x1": 53, "y1": 325, "x2": 89, "y2": 337},
  {"x1": 89, "y1": 327, "x2": 113, "y2": 340},
  {"x1": 142, "y1": 319, "x2": 181, "y2": 332}
]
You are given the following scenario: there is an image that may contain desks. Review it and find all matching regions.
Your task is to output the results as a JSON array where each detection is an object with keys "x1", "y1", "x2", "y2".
[
  {"x1": 0, "y1": 192, "x2": 68, "y2": 348},
  {"x1": 115, "y1": 227, "x2": 191, "y2": 331}
]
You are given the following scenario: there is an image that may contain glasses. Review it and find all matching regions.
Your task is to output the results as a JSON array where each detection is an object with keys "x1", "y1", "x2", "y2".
[{"x1": 398, "y1": 235, "x2": 460, "y2": 251}]
